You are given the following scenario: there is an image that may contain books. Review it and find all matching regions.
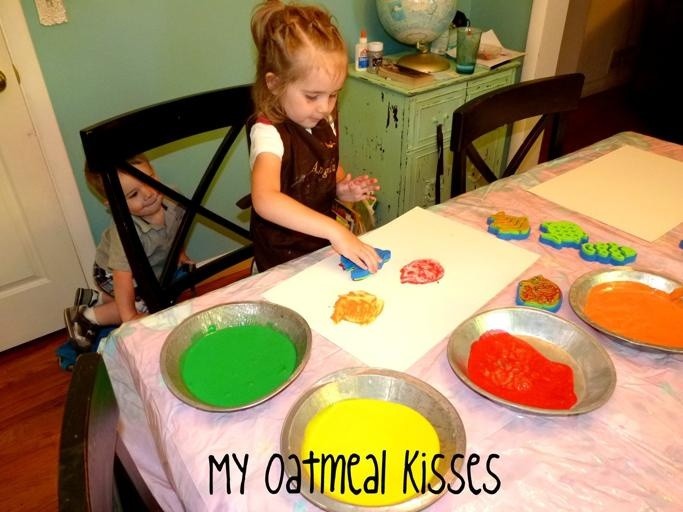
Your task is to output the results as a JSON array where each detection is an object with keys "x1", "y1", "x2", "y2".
[{"x1": 443, "y1": 26, "x2": 528, "y2": 72}]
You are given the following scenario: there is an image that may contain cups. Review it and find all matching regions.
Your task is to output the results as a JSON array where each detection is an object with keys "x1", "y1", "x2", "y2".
[
  {"x1": 455, "y1": 27, "x2": 483, "y2": 74},
  {"x1": 431, "y1": 25, "x2": 449, "y2": 55}
]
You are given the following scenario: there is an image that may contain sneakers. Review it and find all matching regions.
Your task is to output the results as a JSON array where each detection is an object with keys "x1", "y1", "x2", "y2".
[{"x1": 63, "y1": 288, "x2": 99, "y2": 350}]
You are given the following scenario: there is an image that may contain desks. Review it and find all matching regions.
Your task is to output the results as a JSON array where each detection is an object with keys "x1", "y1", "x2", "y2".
[{"x1": 96, "y1": 130, "x2": 681, "y2": 511}]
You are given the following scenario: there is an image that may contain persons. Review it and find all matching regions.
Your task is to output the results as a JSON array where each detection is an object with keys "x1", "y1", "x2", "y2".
[
  {"x1": 246, "y1": 0, "x2": 383, "y2": 274},
  {"x1": 61, "y1": 151, "x2": 189, "y2": 351}
]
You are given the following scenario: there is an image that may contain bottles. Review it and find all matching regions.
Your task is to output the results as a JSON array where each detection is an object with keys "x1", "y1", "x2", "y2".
[
  {"x1": 366, "y1": 41, "x2": 384, "y2": 73},
  {"x1": 354, "y1": 31, "x2": 368, "y2": 72}
]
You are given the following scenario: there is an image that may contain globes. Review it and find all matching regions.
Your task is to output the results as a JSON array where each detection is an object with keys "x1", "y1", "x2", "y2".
[{"x1": 375, "y1": 0, "x2": 458, "y2": 73}]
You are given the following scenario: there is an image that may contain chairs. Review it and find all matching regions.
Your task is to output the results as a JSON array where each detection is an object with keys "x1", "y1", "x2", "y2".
[
  {"x1": 445, "y1": 70, "x2": 585, "y2": 197},
  {"x1": 79, "y1": 83, "x2": 265, "y2": 314},
  {"x1": 56, "y1": 350, "x2": 165, "y2": 512}
]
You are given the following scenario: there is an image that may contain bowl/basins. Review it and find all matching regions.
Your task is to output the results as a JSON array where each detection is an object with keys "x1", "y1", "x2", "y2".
[
  {"x1": 569, "y1": 265, "x2": 683, "y2": 356},
  {"x1": 446, "y1": 307, "x2": 616, "y2": 414},
  {"x1": 277, "y1": 366, "x2": 466, "y2": 511},
  {"x1": 477, "y1": 43, "x2": 502, "y2": 60},
  {"x1": 156, "y1": 301, "x2": 312, "y2": 414}
]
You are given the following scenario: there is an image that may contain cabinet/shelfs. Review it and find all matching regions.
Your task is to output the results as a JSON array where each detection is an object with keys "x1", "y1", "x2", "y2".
[{"x1": 335, "y1": 48, "x2": 522, "y2": 229}]
